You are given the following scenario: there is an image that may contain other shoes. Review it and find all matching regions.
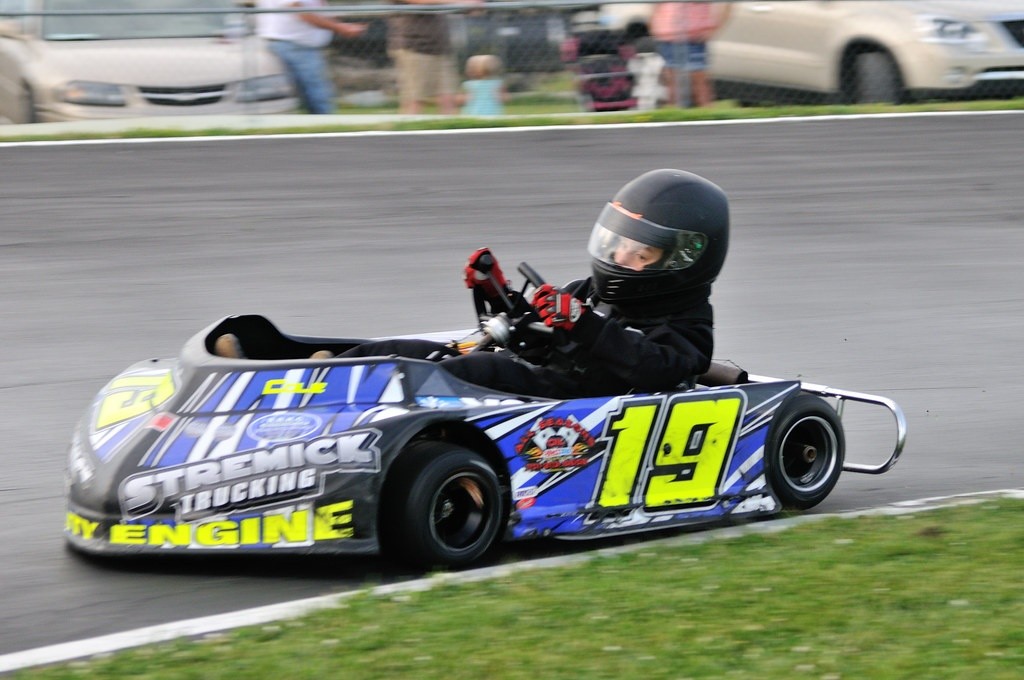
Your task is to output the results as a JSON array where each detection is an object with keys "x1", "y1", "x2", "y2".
[
  {"x1": 311, "y1": 350, "x2": 332, "y2": 359},
  {"x1": 214, "y1": 334, "x2": 245, "y2": 359}
]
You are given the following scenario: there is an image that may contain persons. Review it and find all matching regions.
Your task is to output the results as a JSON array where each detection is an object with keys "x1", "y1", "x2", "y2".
[
  {"x1": 454, "y1": 55, "x2": 511, "y2": 116},
  {"x1": 384, "y1": 0, "x2": 487, "y2": 115},
  {"x1": 216, "y1": 169, "x2": 730, "y2": 399},
  {"x1": 647, "y1": 0, "x2": 733, "y2": 110},
  {"x1": 257, "y1": 1, "x2": 367, "y2": 113}
]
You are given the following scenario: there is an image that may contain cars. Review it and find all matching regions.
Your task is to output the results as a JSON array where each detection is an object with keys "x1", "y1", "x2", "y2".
[
  {"x1": 701, "y1": 0, "x2": 1024, "y2": 111},
  {"x1": 0, "y1": 1, "x2": 300, "y2": 129}
]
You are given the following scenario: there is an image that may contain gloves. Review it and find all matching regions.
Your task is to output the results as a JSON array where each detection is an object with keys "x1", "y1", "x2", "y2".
[
  {"x1": 531, "y1": 284, "x2": 587, "y2": 331},
  {"x1": 464, "y1": 247, "x2": 506, "y2": 298}
]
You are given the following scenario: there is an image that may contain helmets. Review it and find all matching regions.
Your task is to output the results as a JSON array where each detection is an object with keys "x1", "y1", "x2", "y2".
[{"x1": 588, "y1": 168, "x2": 730, "y2": 302}]
